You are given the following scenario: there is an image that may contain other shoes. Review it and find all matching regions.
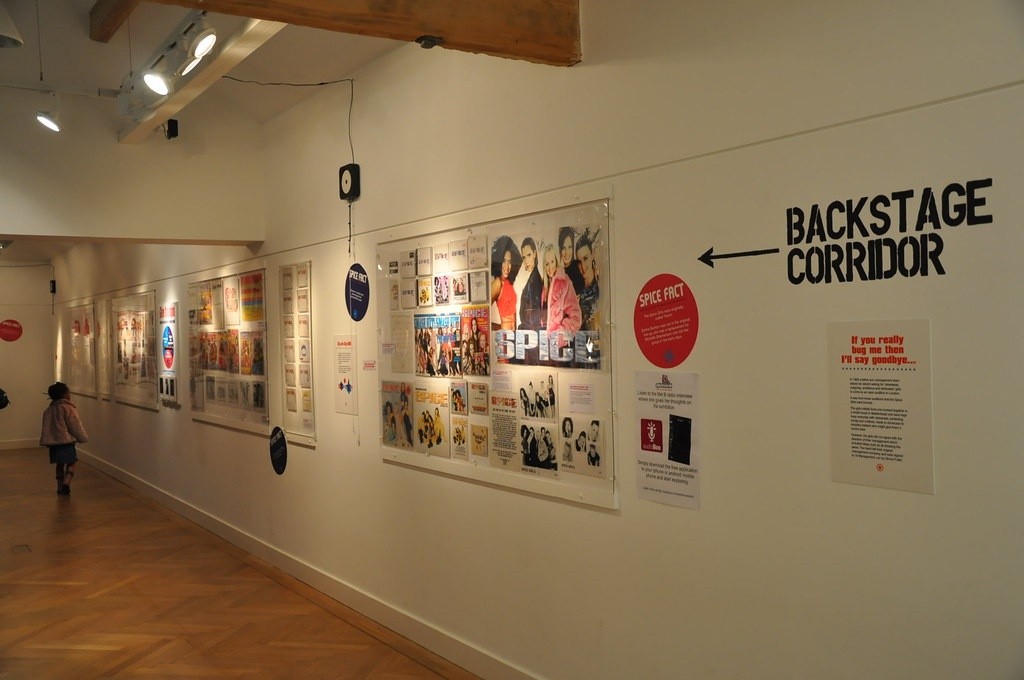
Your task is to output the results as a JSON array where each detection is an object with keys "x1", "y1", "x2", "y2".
[
  {"x1": 62, "y1": 484, "x2": 70, "y2": 495},
  {"x1": 57, "y1": 487, "x2": 63, "y2": 494}
]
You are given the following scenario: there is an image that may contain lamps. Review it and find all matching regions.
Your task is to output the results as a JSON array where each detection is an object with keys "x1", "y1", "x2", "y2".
[{"x1": 0, "y1": 0, "x2": 216, "y2": 133}]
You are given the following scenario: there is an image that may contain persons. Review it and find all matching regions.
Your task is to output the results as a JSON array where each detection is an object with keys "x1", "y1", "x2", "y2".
[
  {"x1": 40, "y1": 380, "x2": 90, "y2": 501},
  {"x1": 379, "y1": 225, "x2": 609, "y2": 477}
]
